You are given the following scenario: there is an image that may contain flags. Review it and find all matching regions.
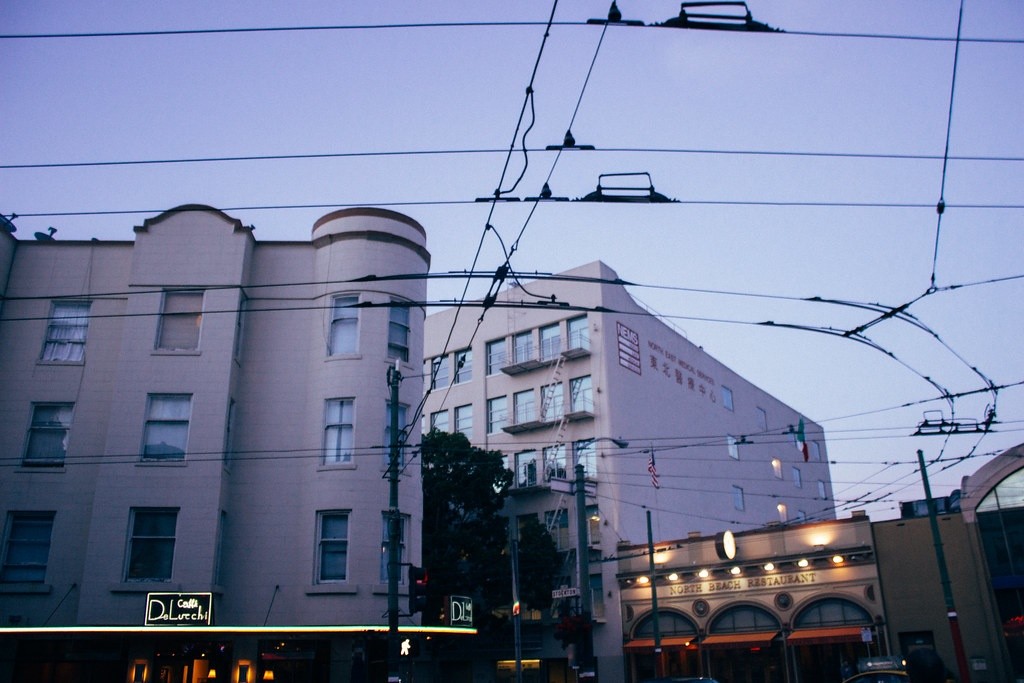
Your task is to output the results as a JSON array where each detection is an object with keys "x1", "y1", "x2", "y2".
[{"x1": 648, "y1": 447, "x2": 660, "y2": 490}]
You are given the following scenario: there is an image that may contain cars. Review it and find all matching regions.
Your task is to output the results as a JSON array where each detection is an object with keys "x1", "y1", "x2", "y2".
[
  {"x1": 840, "y1": 667, "x2": 958, "y2": 683},
  {"x1": 638, "y1": 675, "x2": 720, "y2": 683}
]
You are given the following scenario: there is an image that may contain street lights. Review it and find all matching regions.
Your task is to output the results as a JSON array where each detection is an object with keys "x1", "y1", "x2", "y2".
[{"x1": 571, "y1": 434, "x2": 630, "y2": 683}]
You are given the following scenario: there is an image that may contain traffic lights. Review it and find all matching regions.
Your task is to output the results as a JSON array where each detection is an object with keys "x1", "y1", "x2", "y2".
[{"x1": 408, "y1": 565, "x2": 428, "y2": 613}]
